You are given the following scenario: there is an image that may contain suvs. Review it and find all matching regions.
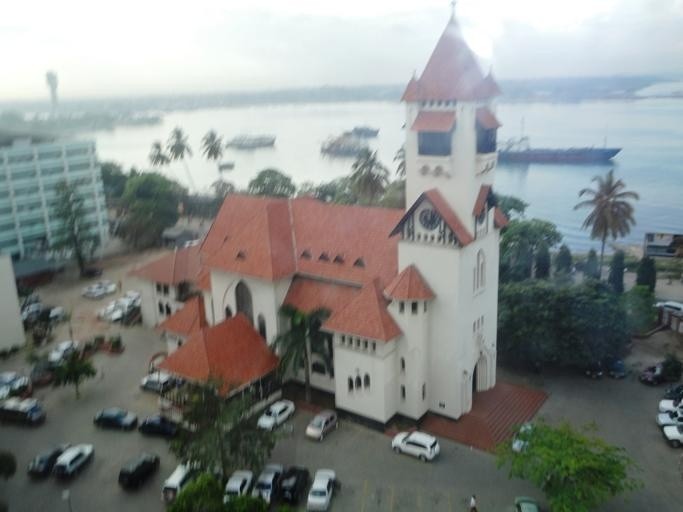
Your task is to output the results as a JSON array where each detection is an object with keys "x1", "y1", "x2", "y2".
[{"x1": 391, "y1": 431, "x2": 440, "y2": 463}]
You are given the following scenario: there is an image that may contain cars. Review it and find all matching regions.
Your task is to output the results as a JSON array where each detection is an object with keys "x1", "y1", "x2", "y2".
[
  {"x1": 0, "y1": 279, "x2": 140, "y2": 431},
  {"x1": 608, "y1": 357, "x2": 625, "y2": 380},
  {"x1": 512, "y1": 496, "x2": 540, "y2": 512},
  {"x1": 25, "y1": 445, "x2": 62, "y2": 479},
  {"x1": 255, "y1": 398, "x2": 296, "y2": 433},
  {"x1": 305, "y1": 408, "x2": 339, "y2": 442},
  {"x1": 93, "y1": 406, "x2": 138, "y2": 431},
  {"x1": 655, "y1": 385, "x2": 683, "y2": 448},
  {"x1": 160, "y1": 461, "x2": 202, "y2": 506},
  {"x1": 251, "y1": 463, "x2": 284, "y2": 510},
  {"x1": 639, "y1": 361, "x2": 682, "y2": 387},
  {"x1": 585, "y1": 356, "x2": 604, "y2": 380},
  {"x1": 118, "y1": 451, "x2": 161, "y2": 492},
  {"x1": 51, "y1": 443, "x2": 95, "y2": 480},
  {"x1": 511, "y1": 422, "x2": 540, "y2": 457},
  {"x1": 222, "y1": 469, "x2": 255, "y2": 506},
  {"x1": 137, "y1": 414, "x2": 180, "y2": 438},
  {"x1": 276, "y1": 465, "x2": 310, "y2": 507},
  {"x1": 306, "y1": 467, "x2": 336, "y2": 512}
]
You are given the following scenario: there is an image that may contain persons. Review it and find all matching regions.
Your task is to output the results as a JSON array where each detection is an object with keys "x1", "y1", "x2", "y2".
[
  {"x1": 148, "y1": 359, "x2": 155, "y2": 374},
  {"x1": 470, "y1": 493, "x2": 478, "y2": 512},
  {"x1": 117, "y1": 278, "x2": 122, "y2": 292}
]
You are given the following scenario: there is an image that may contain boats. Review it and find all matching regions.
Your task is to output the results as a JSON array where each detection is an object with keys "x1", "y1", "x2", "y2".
[
  {"x1": 226, "y1": 134, "x2": 276, "y2": 149},
  {"x1": 497, "y1": 160, "x2": 618, "y2": 189},
  {"x1": 343, "y1": 126, "x2": 381, "y2": 139},
  {"x1": 321, "y1": 143, "x2": 369, "y2": 156},
  {"x1": 498, "y1": 113, "x2": 622, "y2": 162}
]
